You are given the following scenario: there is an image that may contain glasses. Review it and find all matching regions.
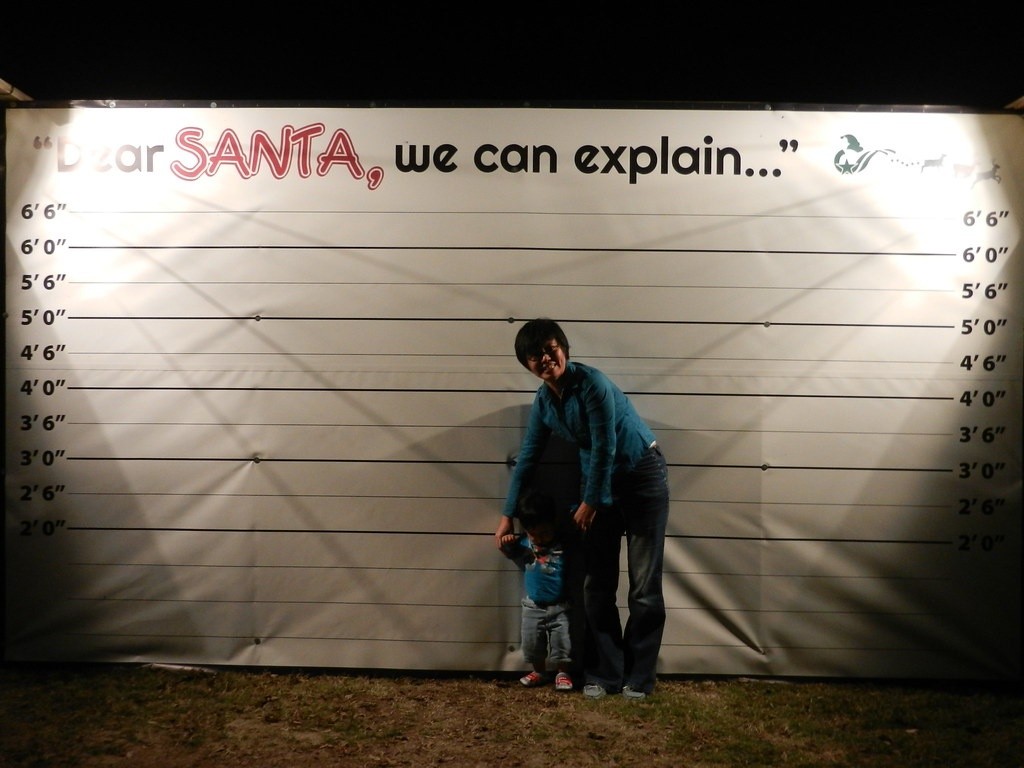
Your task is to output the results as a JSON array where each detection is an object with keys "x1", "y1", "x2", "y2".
[{"x1": 525, "y1": 344, "x2": 561, "y2": 366}]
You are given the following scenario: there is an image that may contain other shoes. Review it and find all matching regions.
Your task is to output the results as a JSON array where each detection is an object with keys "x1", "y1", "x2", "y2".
[
  {"x1": 621, "y1": 685, "x2": 646, "y2": 706},
  {"x1": 583, "y1": 684, "x2": 607, "y2": 701}
]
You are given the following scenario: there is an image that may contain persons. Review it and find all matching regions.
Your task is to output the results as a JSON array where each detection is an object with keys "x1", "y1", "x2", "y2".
[
  {"x1": 495, "y1": 318, "x2": 670, "y2": 698},
  {"x1": 501, "y1": 491, "x2": 586, "y2": 691}
]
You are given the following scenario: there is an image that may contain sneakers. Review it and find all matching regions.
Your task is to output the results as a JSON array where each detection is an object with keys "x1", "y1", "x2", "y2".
[
  {"x1": 555, "y1": 673, "x2": 573, "y2": 689},
  {"x1": 520, "y1": 671, "x2": 551, "y2": 687}
]
